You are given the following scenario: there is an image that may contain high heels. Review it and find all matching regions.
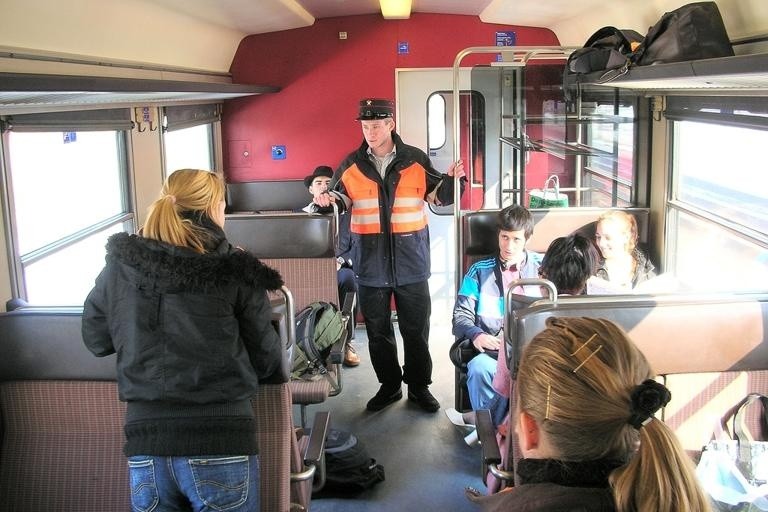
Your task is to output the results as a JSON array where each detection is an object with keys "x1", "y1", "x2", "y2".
[{"x1": 445, "y1": 408, "x2": 481, "y2": 449}]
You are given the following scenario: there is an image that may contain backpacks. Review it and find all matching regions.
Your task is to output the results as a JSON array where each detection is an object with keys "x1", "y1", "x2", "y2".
[
  {"x1": 293, "y1": 297, "x2": 344, "y2": 388},
  {"x1": 295, "y1": 427, "x2": 386, "y2": 496}
]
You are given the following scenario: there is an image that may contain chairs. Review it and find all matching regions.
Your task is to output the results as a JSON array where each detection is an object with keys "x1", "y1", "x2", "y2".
[
  {"x1": 469, "y1": 406, "x2": 513, "y2": 494},
  {"x1": 514, "y1": 296, "x2": 767, "y2": 512},
  {"x1": 285, "y1": 410, "x2": 330, "y2": 496},
  {"x1": 0, "y1": 310, "x2": 294, "y2": 512},
  {"x1": 220, "y1": 176, "x2": 338, "y2": 215},
  {"x1": 451, "y1": 207, "x2": 661, "y2": 411},
  {"x1": 218, "y1": 215, "x2": 349, "y2": 386}
]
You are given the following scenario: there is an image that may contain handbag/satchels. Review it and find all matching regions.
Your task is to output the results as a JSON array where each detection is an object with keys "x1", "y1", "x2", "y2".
[
  {"x1": 694, "y1": 393, "x2": 768, "y2": 512},
  {"x1": 564, "y1": 27, "x2": 645, "y2": 103},
  {"x1": 528, "y1": 176, "x2": 568, "y2": 207},
  {"x1": 449, "y1": 336, "x2": 499, "y2": 373},
  {"x1": 596, "y1": 1, "x2": 736, "y2": 85}
]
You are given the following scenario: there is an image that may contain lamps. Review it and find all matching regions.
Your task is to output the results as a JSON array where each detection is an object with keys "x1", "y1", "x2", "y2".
[{"x1": 379, "y1": 1, "x2": 413, "y2": 19}]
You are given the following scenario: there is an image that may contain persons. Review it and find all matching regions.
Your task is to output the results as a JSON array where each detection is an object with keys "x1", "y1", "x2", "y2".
[
  {"x1": 82, "y1": 168, "x2": 280, "y2": 511},
  {"x1": 445, "y1": 232, "x2": 606, "y2": 491},
  {"x1": 293, "y1": 166, "x2": 360, "y2": 368},
  {"x1": 589, "y1": 211, "x2": 659, "y2": 298},
  {"x1": 464, "y1": 316, "x2": 712, "y2": 512},
  {"x1": 312, "y1": 99, "x2": 469, "y2": 413},
  {"x1": 451, "y1": 205, "x2": 552, "y2": 426}
]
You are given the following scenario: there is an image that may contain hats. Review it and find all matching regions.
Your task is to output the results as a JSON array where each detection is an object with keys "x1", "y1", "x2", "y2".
[
  {"x1": 304, "y1": 166, "x2": 334, "y2": 188},
  {"x1": 355, "y1": 99, "x2": 393, "y2": 121}
]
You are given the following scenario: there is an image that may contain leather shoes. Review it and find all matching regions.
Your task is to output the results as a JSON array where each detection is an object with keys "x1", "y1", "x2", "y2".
[{"x1": 344, "y1": 342, "x2": 360, "y2": 366}]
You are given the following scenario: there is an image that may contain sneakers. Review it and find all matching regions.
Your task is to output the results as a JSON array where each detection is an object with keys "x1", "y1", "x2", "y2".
[
  {"x1": 367, "y1": 384, "x2": 402, "y2": 411},
  {"x1": 407, "y1": 384, "x2": 441, "y2": 411}
]
